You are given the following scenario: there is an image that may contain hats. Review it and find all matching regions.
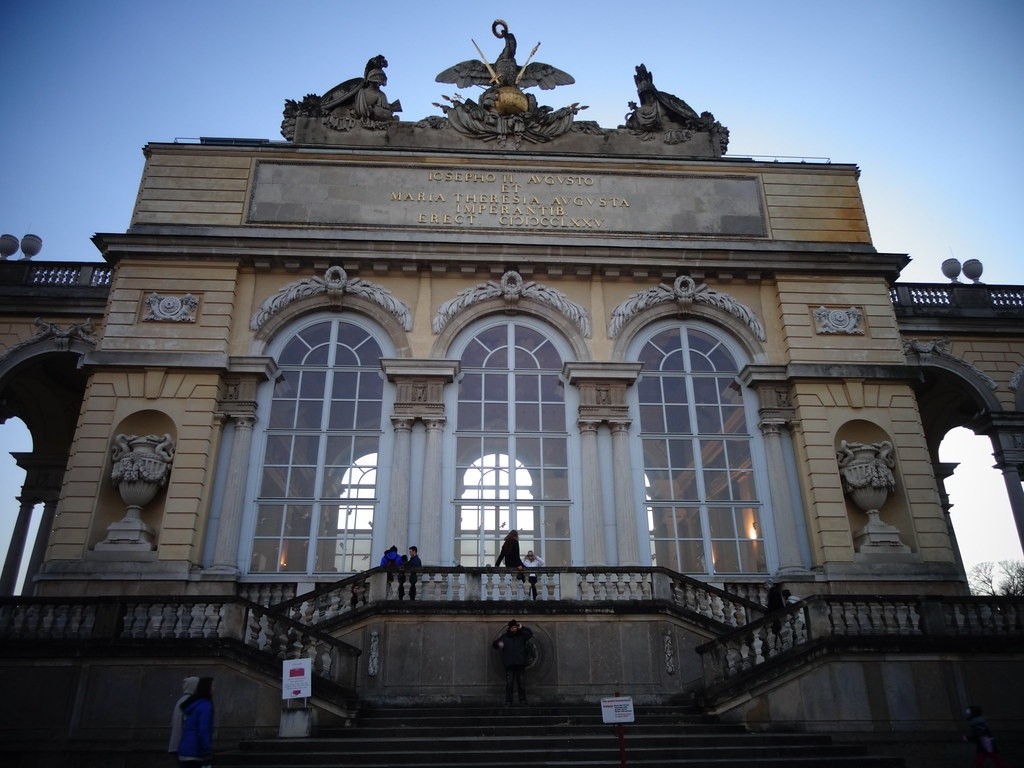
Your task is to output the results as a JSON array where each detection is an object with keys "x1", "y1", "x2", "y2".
[
  {"x1": 390, "y1": 545, "x2": 398, "y2": 553},
  {"x1": 508, "y1": 620, "x2": 517, "y2": 627}
]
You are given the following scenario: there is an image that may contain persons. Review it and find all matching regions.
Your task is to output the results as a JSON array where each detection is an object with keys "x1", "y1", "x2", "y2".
[
  {"x1": 765, "y1": 582, "x2": 806, "y2": 651},
  {"x1": 962, "y1": 706, "x2": 1006, "y2": 768},
  {"x1": 352, "y1": 585, "x2": 359, "y2": 605},
  {"x1": 492, "y1": 619, "x2": 533, "y2": 707},
  {"x1": 168, "y1": 677, "x2": 216, "y2": 768},
  {"x1": 523, "y1": 551, "x2": 545, "y2": 600},
  {"x1": 381, "y1": 545, "x2": 421, "y2": 600},
  {"x1": 495, "y1": 530, "x2": 526, "y2": 584}
]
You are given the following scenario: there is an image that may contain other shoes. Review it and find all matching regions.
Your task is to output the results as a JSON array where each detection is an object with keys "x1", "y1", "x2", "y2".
[
  {"x1": 505, "y1": 702, "x2": 511, "y2": 707},
  {"x1": 521, "y1": 703, "x2": 531, "y2": 707}
]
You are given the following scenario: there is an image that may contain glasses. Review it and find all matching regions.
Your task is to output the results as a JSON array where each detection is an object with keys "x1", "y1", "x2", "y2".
[{"x1": 528, "y1": 555, "x2": 533, "y2": 557}]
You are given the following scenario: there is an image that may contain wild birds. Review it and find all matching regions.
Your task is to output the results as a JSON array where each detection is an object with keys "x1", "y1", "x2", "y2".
[{"x1": 435, "y1": 29, "x2": 575, "y2": 90}]
